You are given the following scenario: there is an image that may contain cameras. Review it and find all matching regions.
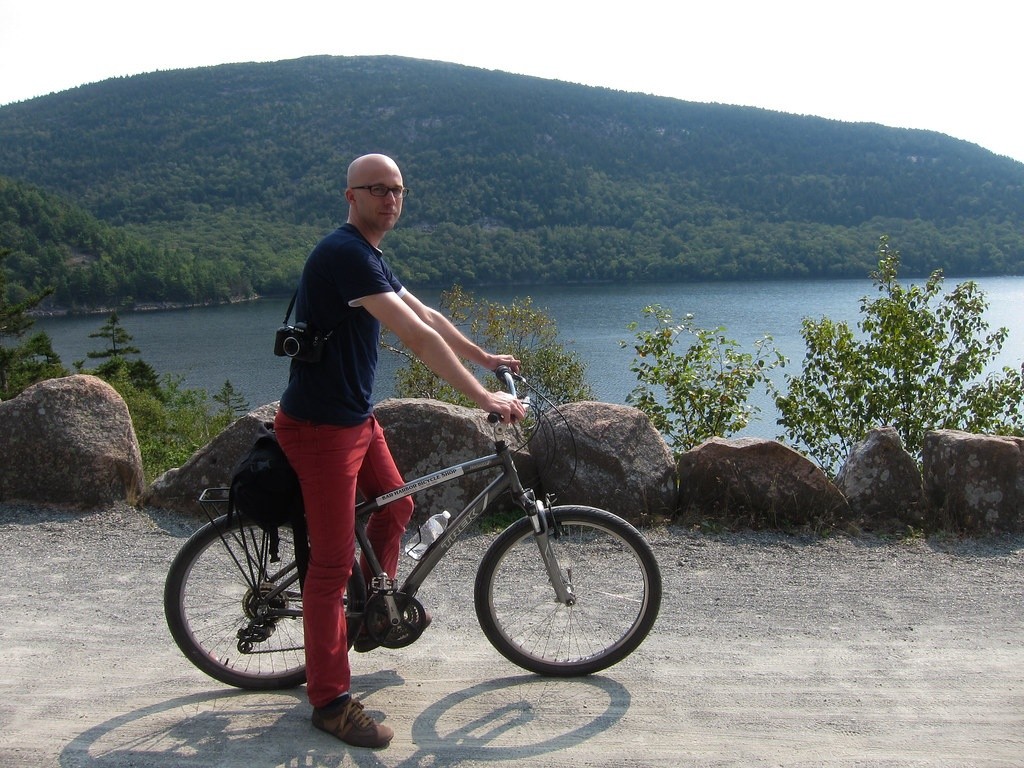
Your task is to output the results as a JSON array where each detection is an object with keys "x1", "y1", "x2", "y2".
[{"x1": 274, "y1": 322, "x2": 325, "y2": 363}]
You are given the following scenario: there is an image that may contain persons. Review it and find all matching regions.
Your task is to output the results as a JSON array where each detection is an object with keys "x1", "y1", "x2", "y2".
[{"x1": 273, "y1": 153, "x2": 525, "y2": 748}]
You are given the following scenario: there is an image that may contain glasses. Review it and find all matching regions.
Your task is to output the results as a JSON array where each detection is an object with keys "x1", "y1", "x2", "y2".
[{"x1": 351, "y1": 185, "x2": 410, "y2": 199}]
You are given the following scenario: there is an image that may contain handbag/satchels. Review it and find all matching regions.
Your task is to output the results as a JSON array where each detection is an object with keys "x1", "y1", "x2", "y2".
[{"x1": 225, "y1": 421, "x2": 305, "y2": 562}]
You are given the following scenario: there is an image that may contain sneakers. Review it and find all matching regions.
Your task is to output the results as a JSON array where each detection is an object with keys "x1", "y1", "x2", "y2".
[
  {"x1": 311, "y1": 694, "x2": 394, "y2": 747},
  {"x1": 353, "y1": 612, "x2": 432, "y2": 653}
]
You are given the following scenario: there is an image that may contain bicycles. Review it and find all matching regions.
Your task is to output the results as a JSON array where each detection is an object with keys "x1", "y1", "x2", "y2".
[{"x1": 160, "y1": 366, "x2": 664, "y2": 693}]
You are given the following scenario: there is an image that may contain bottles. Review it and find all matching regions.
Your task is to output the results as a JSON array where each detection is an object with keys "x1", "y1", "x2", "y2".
[{"x1": 405, "y1": 510, "x2": 451, "y2": 561}]
